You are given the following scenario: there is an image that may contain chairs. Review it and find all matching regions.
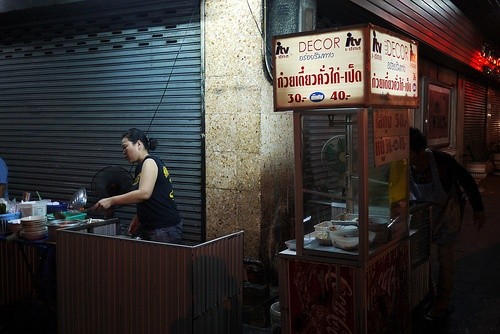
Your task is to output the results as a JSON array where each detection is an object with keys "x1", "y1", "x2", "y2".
[{"x1": 199, "y1": 256, "x2": 278, "y2": 328}]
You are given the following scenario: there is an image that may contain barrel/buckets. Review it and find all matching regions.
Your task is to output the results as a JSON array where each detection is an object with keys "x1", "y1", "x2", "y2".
[{"x1": 20, "y1": 201, "x2": 47, "y2": 217}]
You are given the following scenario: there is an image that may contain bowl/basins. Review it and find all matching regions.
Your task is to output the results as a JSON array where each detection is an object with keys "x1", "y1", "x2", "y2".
[
  {"x1": 330, "y1": 232, "x2": 376, "y2": 250},
  {"x1": 284, "y1": 240, "x2": 296, "y2": 250},
  {"x1": 314, "y1": 221, "x2": 332, "y2": 245},
  {"x1": 8, "y1": 215, "x2": 48, "y2": 240}
]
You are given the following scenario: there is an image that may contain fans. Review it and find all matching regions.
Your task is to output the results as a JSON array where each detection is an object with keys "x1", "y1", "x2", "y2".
[
  {"x1": 91, "y1": 165, "x2": 135, "y2": 218},
  {"x1": 320, "y1": 135, "x2": 360, "y2": 213}
]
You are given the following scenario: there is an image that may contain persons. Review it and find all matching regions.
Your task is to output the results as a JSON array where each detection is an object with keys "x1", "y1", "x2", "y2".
[
  {"x1": 368, "y1": 121, "x2": 486, "y2": 321},
  {"x1": 88, "y1": 128, "x2": 183, "y2": 245},
  {"x1": 0, "y1": 157, "x2": 9, "y2": 202}
]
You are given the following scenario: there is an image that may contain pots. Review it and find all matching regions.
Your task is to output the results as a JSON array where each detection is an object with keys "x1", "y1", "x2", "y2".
[{"x1": 331, "y1": 217, "x2": 392, "y2": 231}]
